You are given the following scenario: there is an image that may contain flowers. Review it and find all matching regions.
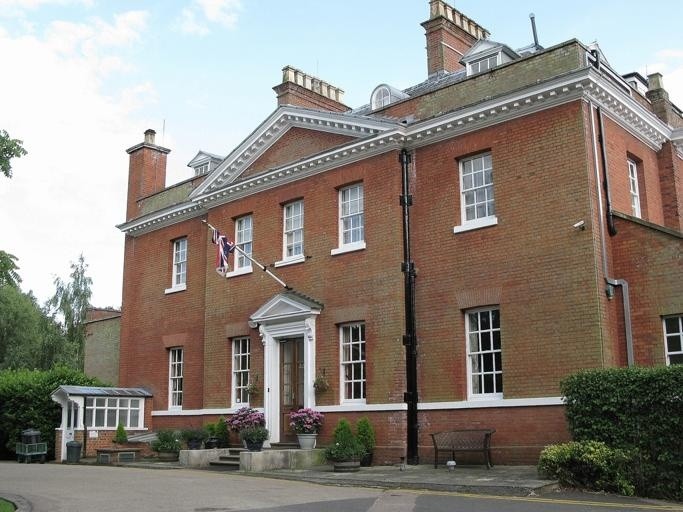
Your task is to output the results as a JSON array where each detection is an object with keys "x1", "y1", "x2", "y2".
[
  {"x1": 289, "y1": 408, "x2": 324, "y2": 434},
  {"x1": 225, "y1": 407, "x2": 266, "y2": 432}
]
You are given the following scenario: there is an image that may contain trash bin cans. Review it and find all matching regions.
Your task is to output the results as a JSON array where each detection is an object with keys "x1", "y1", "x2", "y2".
[{"x1": 66, "y1": 441, "x2": 81, "y2": 465}]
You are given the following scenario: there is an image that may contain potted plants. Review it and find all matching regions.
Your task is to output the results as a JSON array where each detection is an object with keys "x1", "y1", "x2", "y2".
[
  {"x1": 326, "y1": 417, "x2": 363, "y2": 471},
  {"x1": 240, "y1": 427, "x2": 269, "y2": 451},
  {"x1": 355, "y1": 416, "x2": 375, "y2": 467},
  {"x1": 112, "y1": 421, "x2": 129, "y2": 448},
  {"x1": 152, "y1": 407, "x2": 228, "y2": 461}
]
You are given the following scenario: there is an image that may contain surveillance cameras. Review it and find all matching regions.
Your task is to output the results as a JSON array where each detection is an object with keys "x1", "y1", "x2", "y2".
[{"x1": 573, "y1": 221, "x2": 585, "y2": 231}]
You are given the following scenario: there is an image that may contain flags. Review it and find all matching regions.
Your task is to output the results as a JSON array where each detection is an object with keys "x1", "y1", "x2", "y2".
[{"x1": 212, "y1": 229, "x2": 236, "y2": 277}]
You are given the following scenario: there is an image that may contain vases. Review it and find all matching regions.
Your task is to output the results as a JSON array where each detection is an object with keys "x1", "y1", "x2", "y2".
[
  {"x1": 296, "y1": 434, "x2": 319, "y2": 449},
  {"x1": 239, "y1": 433, "x2": 247, "y2": 449}
]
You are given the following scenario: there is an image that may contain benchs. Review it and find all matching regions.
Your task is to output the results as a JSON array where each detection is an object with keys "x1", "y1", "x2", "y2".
[{"x1": 430, "y1": 429, "x2": 495, "y2": 469}]
